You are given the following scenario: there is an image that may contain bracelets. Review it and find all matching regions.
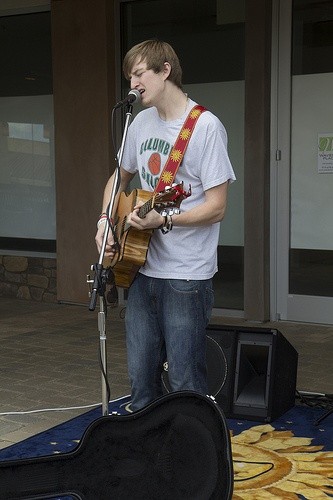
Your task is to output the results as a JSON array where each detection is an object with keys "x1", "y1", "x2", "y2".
[
  {"x1": 155, "y1": 213, "x2": 174, "y2": 235},
  {"x1": 96, "y1": 212, "x2": 116, "y2": 229}
]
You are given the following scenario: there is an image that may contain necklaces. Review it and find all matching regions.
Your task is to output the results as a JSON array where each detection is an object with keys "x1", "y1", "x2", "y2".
[{"x1": 183, "y1": 91, "x2": 191, "y2": 115}]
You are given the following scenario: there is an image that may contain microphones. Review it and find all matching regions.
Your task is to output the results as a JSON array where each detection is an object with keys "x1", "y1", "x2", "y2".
[{"x1": 113, "y1": 89, "x2": 141, "y2": 108}]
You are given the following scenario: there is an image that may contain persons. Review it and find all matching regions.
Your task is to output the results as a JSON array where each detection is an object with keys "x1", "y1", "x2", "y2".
[{"x1": 94, "y1": 39, "x2": 235, "y2": 418}]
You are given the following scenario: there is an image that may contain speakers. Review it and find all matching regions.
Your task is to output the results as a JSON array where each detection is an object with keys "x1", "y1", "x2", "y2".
[{"x1": 158, "y1": 324, "x2": 299, "y2": 422}]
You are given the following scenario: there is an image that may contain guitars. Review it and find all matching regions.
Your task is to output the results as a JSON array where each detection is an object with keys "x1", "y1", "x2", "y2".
[{"x1": 102, "y1": 180, "x2": 193, "y2": 289}]
